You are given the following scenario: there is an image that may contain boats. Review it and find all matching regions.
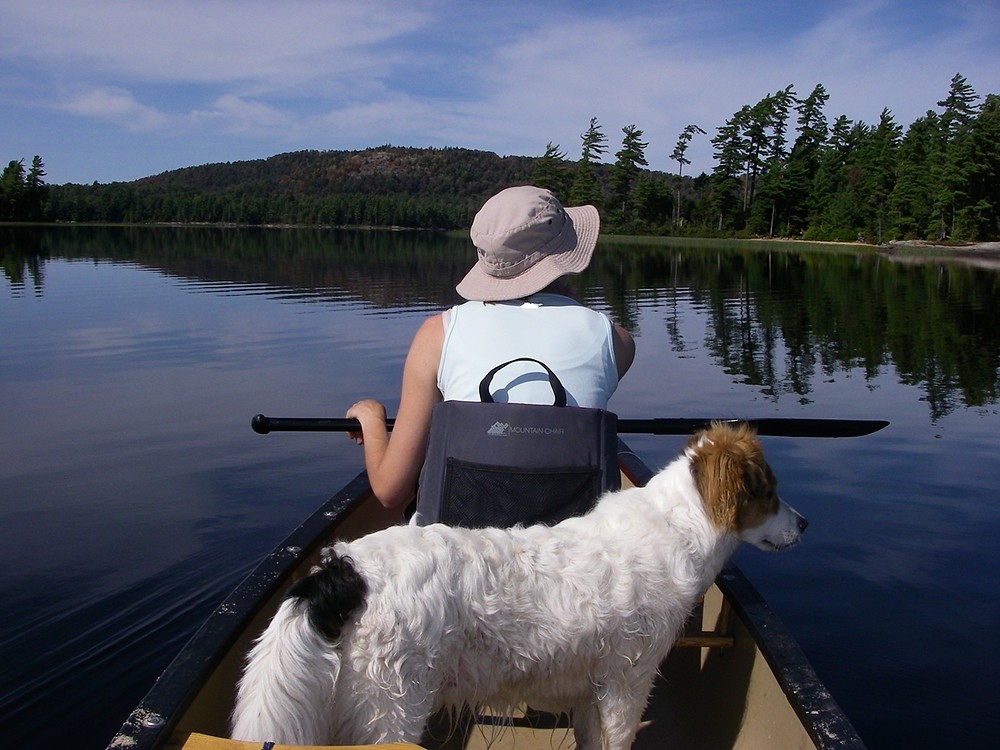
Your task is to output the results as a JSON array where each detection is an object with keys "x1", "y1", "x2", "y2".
[{"x1": 106, "y1": 433, "x2": 868, "y2": 750}]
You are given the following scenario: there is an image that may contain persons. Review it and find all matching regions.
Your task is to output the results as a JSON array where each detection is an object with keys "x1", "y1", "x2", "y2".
[{"x1": 345, "y1": 185, "x2": 635, "y2": 525}]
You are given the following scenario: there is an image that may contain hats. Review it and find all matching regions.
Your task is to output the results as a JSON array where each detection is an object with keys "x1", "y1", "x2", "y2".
[{"x1": 454, "y1": 185, "x2": 601, "y2": 303}]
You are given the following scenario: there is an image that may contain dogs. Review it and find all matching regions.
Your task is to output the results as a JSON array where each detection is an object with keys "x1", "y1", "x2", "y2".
[{"x1": 226, "y1": 418, "x2": 810, "y2": 750}]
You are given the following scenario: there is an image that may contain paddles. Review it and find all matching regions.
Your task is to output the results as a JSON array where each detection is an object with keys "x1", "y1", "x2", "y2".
[{"x1": 251, "y1": 412, "x2": 892, "y2": 437}]
